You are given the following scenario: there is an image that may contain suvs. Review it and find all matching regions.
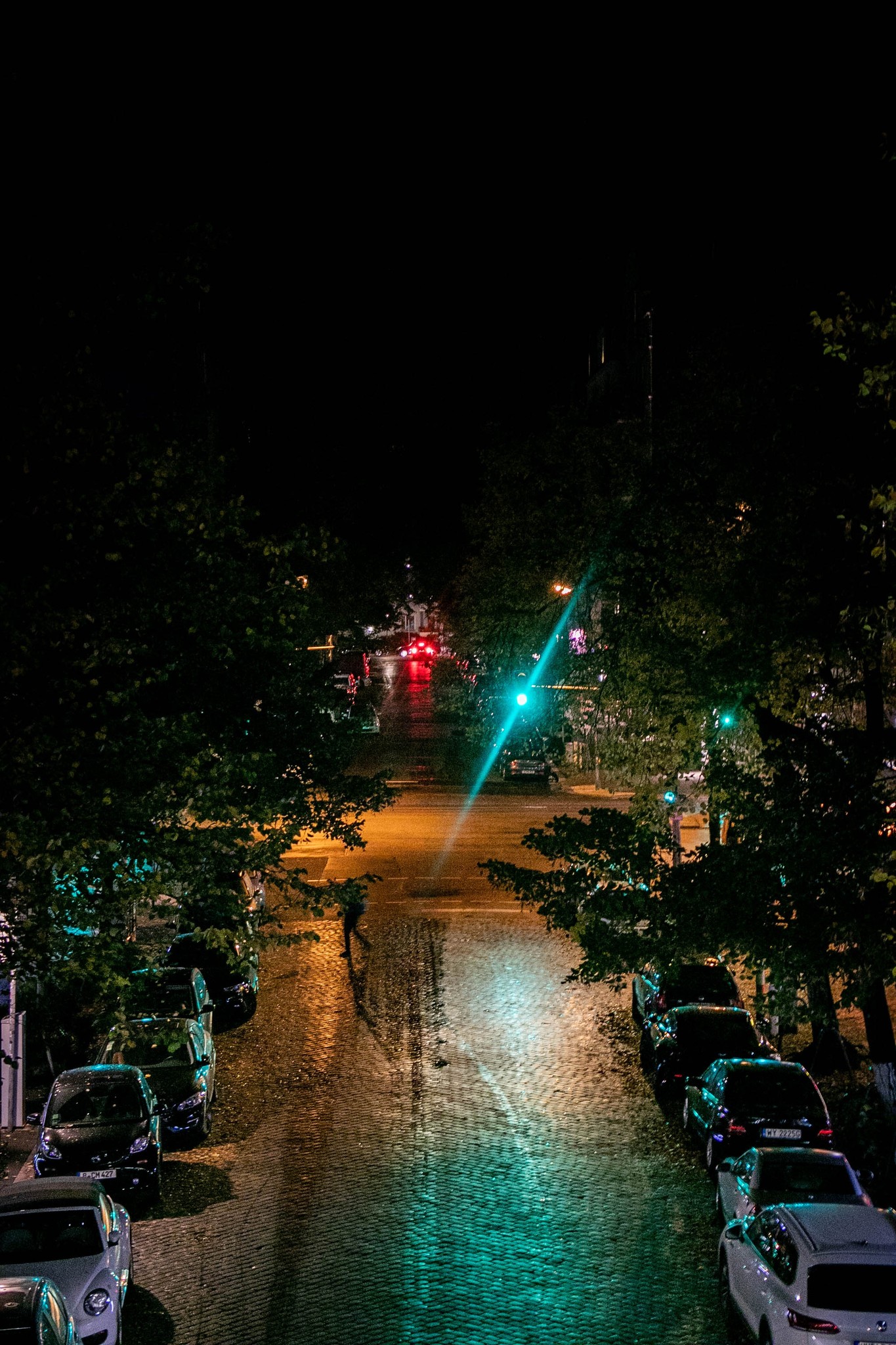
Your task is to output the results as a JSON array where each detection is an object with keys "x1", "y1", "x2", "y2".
[
  {"x1": 368, "y1": 633, "x2": 409, "y2": 656},
  {"x1": 632, "y1": 951, "x2": 743, "y2": 1020},
  {"x1": 720, "y1": 1202, "x2": 896, "y2": 1345}
]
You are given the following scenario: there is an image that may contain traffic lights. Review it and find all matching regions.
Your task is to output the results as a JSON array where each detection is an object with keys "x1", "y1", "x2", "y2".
[
  {"x1": 665, "y1": 791, "x2": 676, "y2": 804},
  {"x1": 719, "y1": 705, "x2": 734, "y2": 727},
  {"x1": 514, "y1": 670, "x2": 530, "y2": 707}
]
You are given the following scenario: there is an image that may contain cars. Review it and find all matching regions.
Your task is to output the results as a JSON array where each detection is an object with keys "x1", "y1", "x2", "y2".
[
  {"x1": 681, "y1": 1058, "x2": 834, "y2": 1172},
  {"x1": 643, "y1": 1005, "x2": 782, "y2": 1098},
  {"x1": 27, "y1": 1064, "x2": 168, "y2": 1196},
  {"x1": 325, "y1": 670, "x2": 381, "y2": 735},
  {"x1": 90, "y1": 1016, "x2": 216, "y2": 1138},
  {"x1": 453, "y1": 656, "x2": 549, "y2": 781},
  {"x1": 114, "y1": 967, "x2": 212, "y2": 1034},
  {"x1": 395, "y1": 639, "x2": 441, "y2": 657},
  {"x1": 159, "y1": 931, "x2": 258, "y2": 1020},
  {"x1": 172, "y1": 849, "x2": 265, "y2": 935},
  {"x1": 716, "y1": 1146, "x2": 873, "y2": 1221},
  {"x1": 0, "y1": 1177, "x2": 131, "y2": 1345}
]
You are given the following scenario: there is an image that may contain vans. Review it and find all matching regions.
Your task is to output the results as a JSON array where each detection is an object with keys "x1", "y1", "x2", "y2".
[{"x1": 339, "y1": 651, "x2": 370, "y2": 679}]
